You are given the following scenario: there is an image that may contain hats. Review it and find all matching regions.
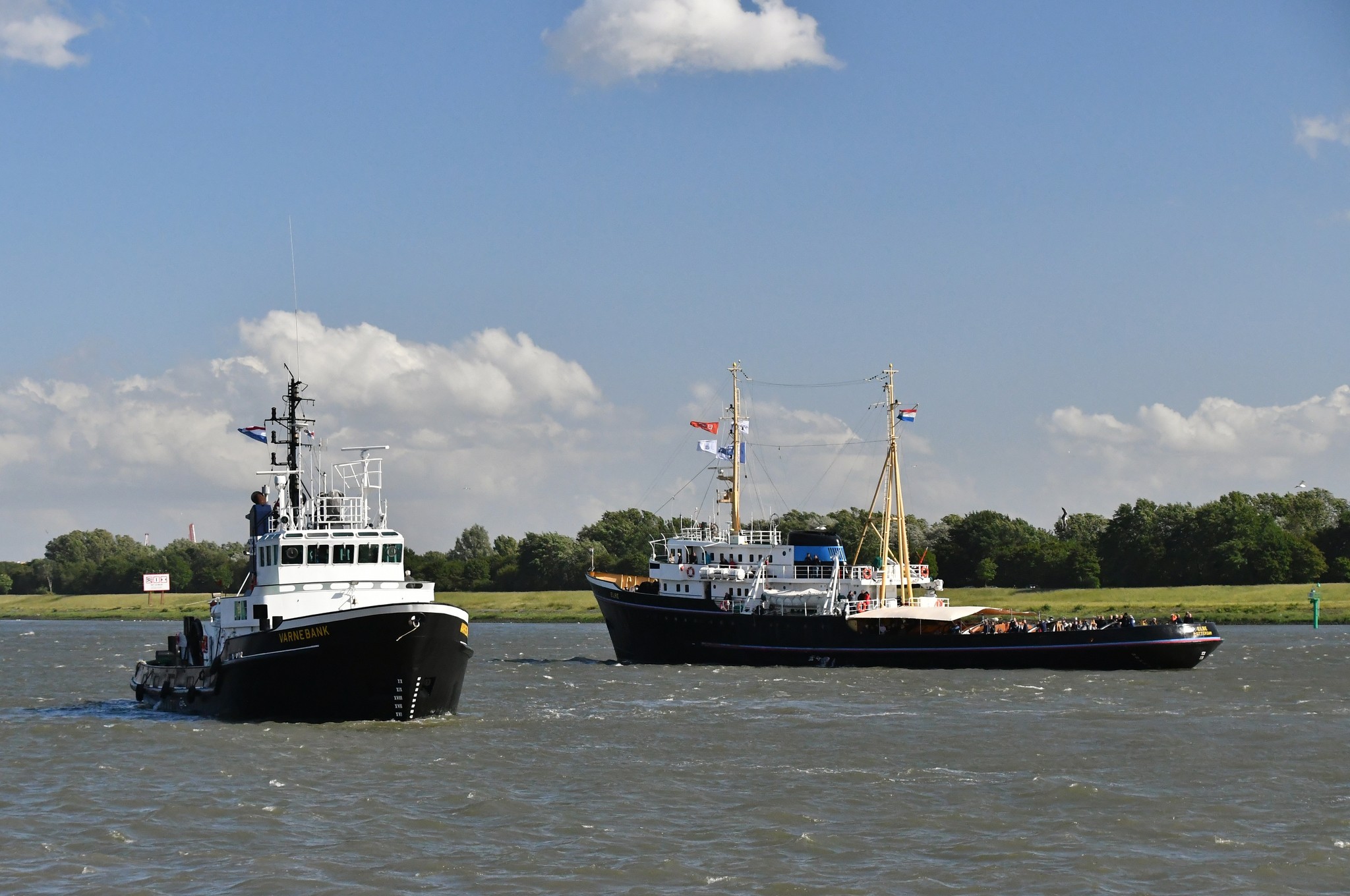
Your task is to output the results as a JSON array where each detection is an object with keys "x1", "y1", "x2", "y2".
[{"x1": 1075, "y1": 617, "x2": 1077, "y2": 620}]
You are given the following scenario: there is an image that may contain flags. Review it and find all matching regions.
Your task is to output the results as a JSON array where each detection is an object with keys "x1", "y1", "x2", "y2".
[
  {"x1": 697, "y1": 440, "x2": 717, "y2": 454},
  {"x1": 896, "y1": 409, "x2": 917, "y2": 422},
  {"x1": 731, "y1": 420, "x2": 749, "y2": 434},
  {"x1": 237, "y1": 426, "x2": 268, "y2": 444},
  {"x1": 716, "y1": 441, "x2": 746, "y2": 464},
  {"x1": 303, "y1": 428, "x2": 315, "y2": 440},
  {"x1": 690, "y1": 421, "x2": 719, "y2": 435}
]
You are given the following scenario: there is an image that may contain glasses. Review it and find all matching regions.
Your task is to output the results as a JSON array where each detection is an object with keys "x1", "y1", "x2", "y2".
[{"x1": 991, "y1": 623, "x2": 993, "y2": 624}]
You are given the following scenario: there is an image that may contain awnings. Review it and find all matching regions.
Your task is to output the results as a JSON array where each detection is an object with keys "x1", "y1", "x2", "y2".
[{"x1": 847, "y1": 606, "x2": 1053, "y2": 635}]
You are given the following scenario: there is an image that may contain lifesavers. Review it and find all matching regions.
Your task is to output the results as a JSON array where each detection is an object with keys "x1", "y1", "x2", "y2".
[
  {"x1": 202, "y1": 636, "x2": 208, "y2": 652},
  {"x1": 188, "y1": 685, "x2": 196, "y2": 704},
  {"x1": 136, "y1": 684, "x2": 143, "y2": 701},
  {"x1": 864, "y1": 568, "x2": 871, "y2": 579},
  {"x1": 160, "y1": 681, "x2": 169, "y2": 699},
  {"x1": 720, "y1": 601, "x2": 730, "y2": 611},
  {"x1": 921, "y1": 566, "x2": 929, "y2": 577},
  {"x1": 858, "y1": 601, "x2": 866, "y2": 613},
  {"x1": 687, "y1": 567, "x2": 694, "y2": 577},
  {"x1": 250, "y1": 572, "x2": 256, "y2": 591},
  {"x1": 937, "y1": 599, "x2": 943, "y2": 606}
]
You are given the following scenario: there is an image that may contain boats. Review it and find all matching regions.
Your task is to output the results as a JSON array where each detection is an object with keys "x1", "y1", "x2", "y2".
[
  {"x1": 586, "y1": 361, "x2": 1226, "y2": 674},
  {"x1": 131, "y1": 361, "x2": 476, "y2": 726}
]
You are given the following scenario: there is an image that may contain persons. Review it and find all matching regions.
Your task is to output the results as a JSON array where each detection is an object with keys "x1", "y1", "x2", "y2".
[
  {"x1": 1142, "y1": 619, "x2": 1148, "y2": 625},
  {"x1": 1166, "y1": 611, "x2": 1194, "y2": 626},
  {"x1": 860, "y1": 619, "x2": 910, "y2": 636},
  {"x1": 1036, "y1": 611, "x2": 1135, "y2": 632},
  {"x1": 1150, "y1": 618, "x2": 1157, "y2": 625},
  {"x1": 723, "y1": 593, "x2": 733, "y2": 612},
  {"x1": 653, "y1": 579, "x2": 659, "y2": 595},
  {"x1": 669, "y1": 553, "x2": 675, "y2": 563},
  {"x1": 677, "y1": 553, "x2": 682, "y2": 564},
  {"x1": 761, "y1": 592, "x2": 766, "y2": 605},
  {"x1": 691, "y1": 552, "x2": 698, "y2": 564},
  {"x1": 1006, "y1": 617, "x2": 1028, "y2": 633},
  {"x1": 980, "y1": 620, "x2": 996, "y2": 633},
  {"x1": 805, "y1": 553, "x2": 821, "y2": 578},
  {"x1": 703, "y1": 551, "x2": 711, "y2": 565},
  {"x1": 729, "y1": 558, "x2": 737, "y2": 568},
  {"x1": 952, "y1": 623, "x2": 960, "y2": 634},
  {"x1": 719, "y1": 558, "x2": 729, "y2": 568},
  {"x1": 847, "y1": 590, "x2": 871, "y2": 615}
]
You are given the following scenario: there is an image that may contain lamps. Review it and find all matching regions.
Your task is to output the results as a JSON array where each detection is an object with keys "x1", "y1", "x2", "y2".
[
  {"x1": 289, "y1": 453, "x2": 292, "y2": 461},
  {"x1": 294, "y1": 387, "x2": 299, "y2": 398},
  {"x1": 295, "y1": 432, "x2": 299, "y2": 439},
  {"x1": 351, "y1": 581, "x2": 358, "y2": 585},
  {"x1": 271, "y1": 407, "x2": 277, "y2": 419},
  {"x1": 377, "y1": 513, "x2": 388, "y2": 529},
  {"x1": 295, "y1": 478, "x2": 299, "y2": 489},
  {"x1": 271, "y1": 452, "x2": 276, "y2": 464},
  {"x1": 271, "y1": 431, "x2": 276, "y2": 443},
  {"x1": 281, "y1": 515, "x2": 290, "y2": 531}
]
